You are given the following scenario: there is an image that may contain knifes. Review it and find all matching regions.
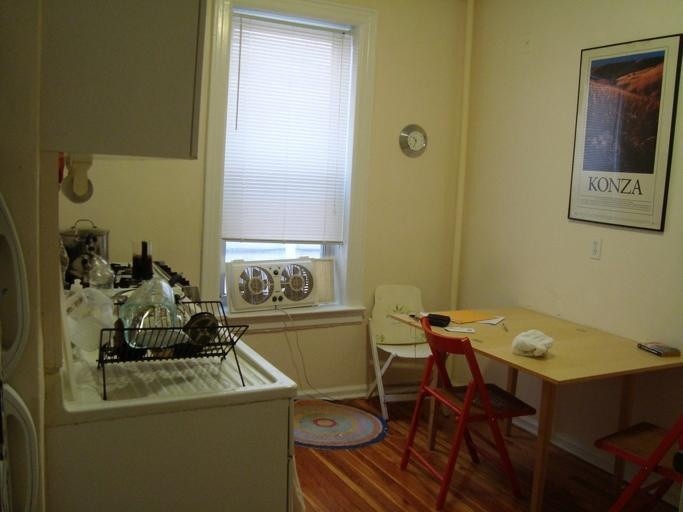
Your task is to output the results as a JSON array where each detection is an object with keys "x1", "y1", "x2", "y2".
[{"x1": 140, "y1": 240, "x2": 148, "y2": 259}]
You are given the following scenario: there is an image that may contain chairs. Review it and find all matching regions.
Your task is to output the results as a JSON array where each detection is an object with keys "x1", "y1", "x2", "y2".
[
  {"x1": 399, "y1": 317, "x2": 536, "y2": 510},
  {"x1": 368, "y1": 284, "x2": 453, "y2": 421},
  {"x1": 595, "y1": 418, "x2": 682, "y2": 511}
]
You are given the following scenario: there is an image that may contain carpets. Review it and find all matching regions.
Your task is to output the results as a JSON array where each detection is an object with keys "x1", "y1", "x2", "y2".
[{"x1": 290, "y1": 399, "x2": 389, "y2": 449}]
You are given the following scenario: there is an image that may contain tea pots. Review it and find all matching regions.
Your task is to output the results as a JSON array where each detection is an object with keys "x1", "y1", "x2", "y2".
[{"x1": 66, "y1": 234, "x2": 115, "y2": 288}]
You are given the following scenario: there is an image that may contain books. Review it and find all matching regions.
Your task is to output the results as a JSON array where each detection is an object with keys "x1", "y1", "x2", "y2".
[{"x1": 638, "y1": 342, "x2": 680, "y2": 357}]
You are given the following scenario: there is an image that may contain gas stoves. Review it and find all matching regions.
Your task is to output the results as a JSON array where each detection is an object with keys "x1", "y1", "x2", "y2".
[{"x1": 113, "y1": 263, "x2": 143, "y2": 289}]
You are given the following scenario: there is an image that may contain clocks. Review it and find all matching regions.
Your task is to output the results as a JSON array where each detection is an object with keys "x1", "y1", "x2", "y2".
[{"x1": 399, "y1": 124, "x2": 428, "y2": 156}]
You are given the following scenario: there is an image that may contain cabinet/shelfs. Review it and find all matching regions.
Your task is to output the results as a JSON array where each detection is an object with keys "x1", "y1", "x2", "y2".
[{"x1": 0, "y1": 1, "x2": 205, "y2": 161}]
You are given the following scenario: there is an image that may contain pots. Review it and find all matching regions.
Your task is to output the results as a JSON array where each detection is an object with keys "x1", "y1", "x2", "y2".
[{"x1": 60, "y1": 220, "x2": 108, "y2": 263}]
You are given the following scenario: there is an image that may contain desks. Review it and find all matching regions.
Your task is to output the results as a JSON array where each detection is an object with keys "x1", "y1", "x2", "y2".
[{"x1": 389, "y1": 308, "x2": 681, "y2": 511}]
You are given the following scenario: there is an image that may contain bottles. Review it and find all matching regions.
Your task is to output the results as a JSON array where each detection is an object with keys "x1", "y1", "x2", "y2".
[{"x1": 65, "y1": 278, "x2": 88, "y2": 313}]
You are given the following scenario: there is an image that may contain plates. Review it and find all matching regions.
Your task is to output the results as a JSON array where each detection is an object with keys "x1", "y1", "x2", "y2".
[
  {"x1": 184, "y1": 311, "x2": 219, "y2": 344},
  {"x1": 113, "y1": 290, "x2": 184, "y2": 306}
]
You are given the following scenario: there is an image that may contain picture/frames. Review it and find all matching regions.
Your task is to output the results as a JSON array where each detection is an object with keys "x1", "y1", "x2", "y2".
[{"x1": 567, "y1": 33, "x2": 682, "y2": 233}]
